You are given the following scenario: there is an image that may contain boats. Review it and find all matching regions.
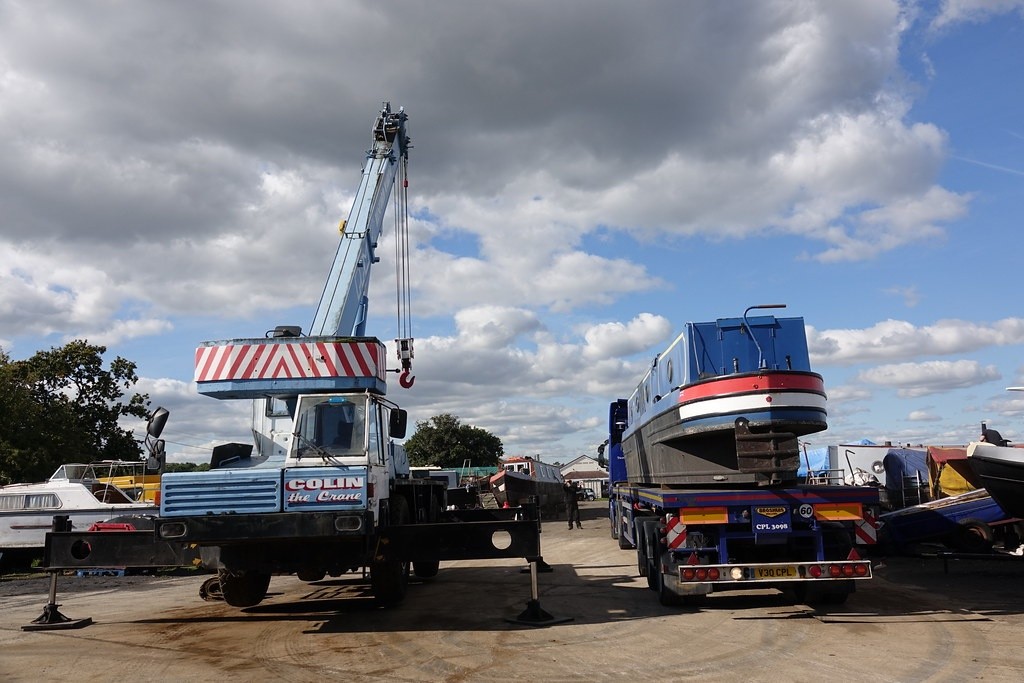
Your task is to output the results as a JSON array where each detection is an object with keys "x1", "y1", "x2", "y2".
[
  {"x1": 0, "y1": 459, "x2": 162, "y2": 552},
  {"x1": 885, "y1": 448, "x2": 929, "y2": 489},
  {"x1": 928, "y1": 445, "x2": 983, "y2": 490},
  {"x1": 966, "y1": 440, "x2": 1024, "y2": 517},
  {"x1": 879, "y1": 486, "x2": 1006, "y2": 540},
  {"x1": 487, "y1": 456, "x2": 568, "y2": 520}
]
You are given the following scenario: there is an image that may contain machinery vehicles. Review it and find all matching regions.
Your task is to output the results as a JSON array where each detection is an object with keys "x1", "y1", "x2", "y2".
[{"x1": 19, "y1": 101, "x2": 585, "y2": 634}]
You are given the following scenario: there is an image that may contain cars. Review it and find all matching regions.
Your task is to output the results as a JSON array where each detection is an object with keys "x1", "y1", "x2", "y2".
[
  {"x1": 584, "y1": 488, "x2": 595, "y2": 501},
  {"x1": 576, "y1": 488, "x2": 587, "y2": 501}
]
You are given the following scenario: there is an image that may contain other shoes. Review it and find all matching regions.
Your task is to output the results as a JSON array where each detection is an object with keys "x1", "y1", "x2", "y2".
[
  {"x1": 578, "y1": 525, "x2": 583, "y2": 529},
  {"x1": 568, "y1": 525, "x2": 573, "y2": 530}
]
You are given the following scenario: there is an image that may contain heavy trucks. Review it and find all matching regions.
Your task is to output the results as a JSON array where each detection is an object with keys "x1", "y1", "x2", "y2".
[{"x1": 596, "y1": 398, "x2": 883, "y2": 611}]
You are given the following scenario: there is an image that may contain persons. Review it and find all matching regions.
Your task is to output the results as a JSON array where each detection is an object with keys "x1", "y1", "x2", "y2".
[{"x1": 563, "y1": 479, "x2": 583, "y2": 531}]
